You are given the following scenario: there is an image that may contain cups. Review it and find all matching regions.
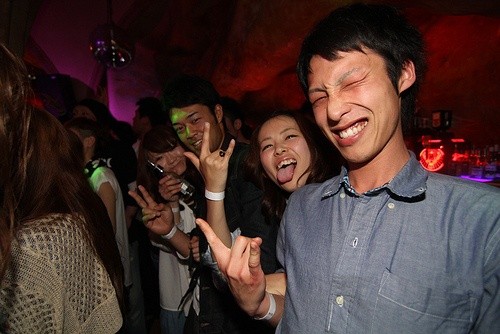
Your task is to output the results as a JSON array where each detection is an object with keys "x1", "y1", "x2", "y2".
[{"x1": 420, "y1": 118, "x2": 432, "y2": 129}]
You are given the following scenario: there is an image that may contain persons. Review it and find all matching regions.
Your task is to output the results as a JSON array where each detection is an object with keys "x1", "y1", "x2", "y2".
[
  {"x1": 129, "y1": 85, "x2": 285, "y2": 333},
  {"x1": 135, "y1": 123, "x2": 205, "y2": 334},
  {"x1": 0, "y1": 43, "x2": 134, "y2": 334},
  {"x1": 196, "y1": 4, "x2": 500, "y2": 334},
  {"x1": 187, "y1": 106, "x2": 344, "y2": 295}
]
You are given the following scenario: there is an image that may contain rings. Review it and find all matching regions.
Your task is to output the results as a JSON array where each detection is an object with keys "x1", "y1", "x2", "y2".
[{"x1": 218, "y1": 150, "x2": 226, "y2": 157}]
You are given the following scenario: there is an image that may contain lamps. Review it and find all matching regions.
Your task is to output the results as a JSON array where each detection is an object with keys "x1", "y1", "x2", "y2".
[{"x1": 88, "y1": 27, "x2": 131, "y2": 70}]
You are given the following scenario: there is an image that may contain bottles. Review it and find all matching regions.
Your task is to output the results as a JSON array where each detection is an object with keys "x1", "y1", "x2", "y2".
[
  {"x1": 420, "y1": 138, "x2": 500, "y2": 182},
  {"x1": 145, "y1": 158, "x2": 197, "y2": 200},
  {"x1": 411, "y1": 98, "x2": 421, "y2": 131}
]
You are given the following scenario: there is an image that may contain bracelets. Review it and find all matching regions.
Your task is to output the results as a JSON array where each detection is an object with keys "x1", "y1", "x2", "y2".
[
  {"x1": 204, "y1": 188, "x2": 225, "y2": 201},
  {"x1": 171, "y1": 206, "x2": 179, "y2": 213},
  {"x1": 255, "y1": 293, "x2": 277, "y2": 321},
  {"x1": 161, "y1": 225, "x2": 178, "y2": 240}
]
